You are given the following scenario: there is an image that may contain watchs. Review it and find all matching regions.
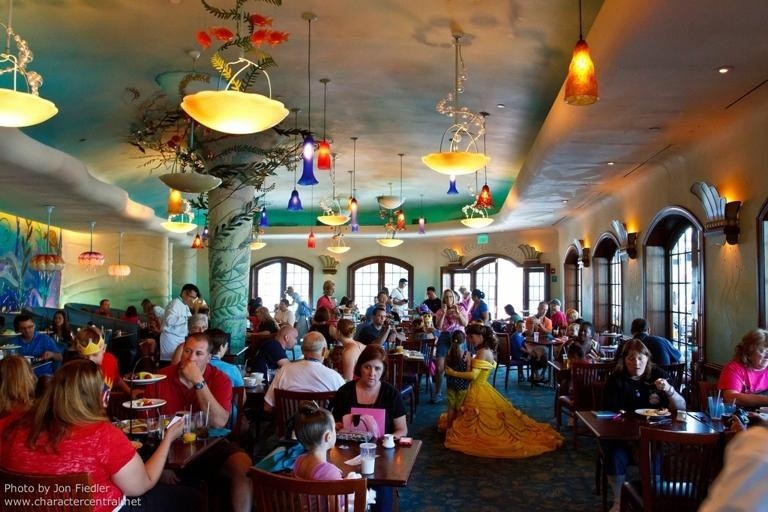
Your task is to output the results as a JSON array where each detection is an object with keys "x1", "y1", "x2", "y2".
[{"x1": 194, "y1": 379, "x2": 205, "y2": 390}]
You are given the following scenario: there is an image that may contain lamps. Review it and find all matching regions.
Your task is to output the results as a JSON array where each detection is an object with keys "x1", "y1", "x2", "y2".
[
  {"x1": 422, "y1": 30, "x2": 490, "y2": 176},
  {"x1": 461, "y1": 172, "x2": 494, "y2": 229},
  {"x1": 572, "y1": 238, "x2": 592, "y2": 268},
  {"x1": 518, "y1": 244, "x2": 541, "y2": 265},
  {"x1": 396, "y1": 153, "x2": 406, "y2": 232},
  {"x1": 299, "y1": 13, "x2": 318, "y2": 188},
  {"x1": 475, "y1": 112, "x2": 494, "y2": 211},
  {"x1": 287, "y1": 109, "x2": 304, "y2": 212},
  {"x1": 441, "y1": 247, "x2": 463, "y2": 268},
  {"x1": 419, "y1": 194, "x2": 425, "y2": 234},
  {"x1": 320, "y1": 255, "x2": 338, "y2": 274},
  {"x1": 181, "y1": 1, "x2": 290, "y2": 136},
  {"x1": 0, "y1": 3, "x2": 59, "y2": 127},
  {"x1": 79, "y1": 221, "x2": 104, "y2": 270},
  {"x1": 349, "y1": 136, "x2": 359, "y2": 232},
  {"x1": 609, "y1": 220, "x2": 638, "y2": 259},
  {"x1": 348, "y1": 171, "x2": 352, "y2": 226},
  {"x1": 448, "y1": 138, "x2": 458, "y2": 196},
  {"x1": 30, "y1": 204, "x2": 65, "y2": 277},
  {"x1": 316, "y1": 78, "x2": 331, "y2": 170},
  {"x1": 308, "y1": 184, "x2": 315, "y2": 249},
  {"x1": 564, "y1": 1, "x2": 602, "y2": 106},
  {"x1": 108, "y1": 231, "x2": 131, "y2": 280},
  {"x1": 260, "y1": 179, "x2": 269, "y2": 228},
  {"x1": 690, "y1": 181, "x2": 742, "y2": 247},
  {"x1": 377, "y1": 183, "x2": 405, "y2": 248}
]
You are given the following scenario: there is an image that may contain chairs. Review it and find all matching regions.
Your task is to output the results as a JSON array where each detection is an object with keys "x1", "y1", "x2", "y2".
[
  {"x1": 1, "y1": 471, "x2": 89, "y2": 511},
  {"x1": 250, "y1": 469, "x2": 369, "y2": 512},
  {"x1": 230, "y1": 386, "x2": 245, "y2": 437},
  {"x1": 660, "y1": 364, "x2": 686, "y2": 387},
  {"x1": 553, "y1": 362, "x2": 614, "y2": 430},
  {"x1": 275, "y1": 388, "x2": 337, "y2": 428},
  {"x1": 493, "y1": 333, "x2": 534, "y2": 391},
  {"x1": 418, "y1": 340, "x2": 436, "y2": 400},
  {"x1": 384, "y1": 355, "x2": 416, "y2": 424}
]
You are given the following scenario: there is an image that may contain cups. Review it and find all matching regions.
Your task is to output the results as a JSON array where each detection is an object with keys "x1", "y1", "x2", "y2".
[
  {"x1": 158, "y1": 415, "x2": 171, "y2": 437},
  {"x1": 267, "y1": 368, "x2": 276, "y2": 382},
  {"x1": 194, "y1": 411, "x2": 210, "y2": 441},
  {"x1": 382, "y1": 434, "x2": 395, "y2": 448},
  {"x1": 359, "y1": 441, "x2": 376, "y2": 476},
  {"x1": 175, "y1": 411, "x2": 191, "y2": 441},
  {"x1": 722, "y1": 402, "x2": 736, "y2": 418},
  {"x1": 533, "y1": 331, "x2": 539, "y2": 342},
  {"x1": 252, "y1": 372, "x2": 265, "y2": 385},
  {"x1": 397, "y1": 345, "x2": 403, "y2": 354},
  {"x1": 563, "y1": 354, "x2": 568, "y2": 367},
  {"x1": 708, "y1": 396, "x2": 725, "y2": 420},
  {"x1": 244, "y1": 376, "x2": 256, "y2": 387},
  {"x1": 182, "y1": 417, "x2": 198, "y2": 444}
]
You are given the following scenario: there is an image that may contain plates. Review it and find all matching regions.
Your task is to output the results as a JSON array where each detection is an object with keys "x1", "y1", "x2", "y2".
[
  {"x1": 634, "y1": 407, "x2": 671, "y2": 419},
  {"x1": 113, "y1": 419, "x2": 168, "y2": 435},
  {"x1": 0, "y1": 344, "x2": 22, "y2": 351},
  {"x1": 123, "y1": 373, "x2": 167, "y2": 386},
  {"x1": 123, "y1": 399, "x2": 167, "y2": 409},
  {"x1": 2, "y1": 332, "x2": 22, "y2": 338}
]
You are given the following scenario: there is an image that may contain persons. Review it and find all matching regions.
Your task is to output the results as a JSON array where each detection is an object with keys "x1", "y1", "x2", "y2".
[
  {"x1": 308, "y1": 307, "x2": 338, "y2": 348},
  {"x1": 510, "y1": 320, "x2": 544, "y2": 382},
  {"x1": 415, "y1": 320, "x2": 440, "y2": 392},
  {"x1": 247, "y1": 306, "x2": 279, "y2": 373},
  {"x1": 366, "y1": 291, "x2": 392, "y2": 322},
  {"x1": 389, "y1": 278, "x2": 408, "y2": 316},
  {"x1": 159, "y1": 283, "x2": 199, "y2": 368},
  {"x1": 411, "y1": 317, "x2": 423, "y2": 340},
  {"x1": 615, "y1": 318, "x2": 681, "y2": 366},
  {"x1": 549, "y1": 299, "x2": 567, "y2": 335},
  {"x1": 1, "y1": 358, "x2": 186, "y2": 512},
  {"x1": 75, "y1": 325, "x2": 144, "y2": 416},
  {"x1": 470, "y1": 289, "x2": 488, "y2": 321},
  {"x1": 133, "y1": 331, "x2": 253, "y2": 512},
  {"x1": 141, "y1": 299, "x2": 165, "y2": 323},
  {"x1": 120, "y1": 305, "x2": 144, "y2": 329},
  {"x1": 254, "y1": 325, "x2": 298, "y2": 383},
  {"x1": 558, "y1": 321, "x2": 605, "y2": 363},
  {"x1": 331, "y1": 350, "x2": 408, "y2": 512},
  {"x1": 263, "y1": 332, "x2": 346, "y2": 437},
  {"x1": 274, "y1": 299, "x2": 296, "y2": 326},
  {"x1": 285, "y1": 286, "x2": 311, "y2": 319},
  {"x1": 559, "y1": 344, "x2": 600, "y2": 426},
  {"x1": 337, "y1": 319, "x2": 366, "y2": 381},
  {"x1": 555, "y1": 323, "x2": 580, "y2": 345},
  {"x1": 454, "y1": 291, "x2": 468, "y2": 311},
  {"x1": 293, "y1": 401, "x2": 370, "y2": 512},
  {"x1": 438, "y1": 318, "x2": 565, "y2": 460},
  {"x1": 0, "y1": 354, "x2": 35, "y2": 432},
  {"x1": 8, "y1": 313, "x2": 62, "y2": 376},
  {"x1": 431, "y1": 288, "x2": 468, "y2": 404},
  {"x1": 360, "y1": 307, "x2": 392, "y2": 352},
  {"x1": 416, "y1": 286, "x2": 441, "y2": 320},
  {"x1": 715, "y1": 328, "x2": 768, "y2": 406},
  {"x1": 95, "y1": 299, "x2": 112, "y2": 316},
  {"x1": 340, "y1": 295, "x2": 360, "y2": 321},
  {"x1": 444, "y1": 330, "x2": 471, "y2": 428},
  {"x1": 730, "y1": 412, "x2": 767, "y2": 432},
  {"x1": 601, "y1": 339, "x2": 686, "y2": 512},
  {"x1": 504, "y1": 304, "x2": 521, "y2": 324},
  {"x1": 566, "y1": 308, "x2": 579, "y2": 324},
  {"x1": 46, "y1": 312, "x2": 75, "y2": 368},
  {"x1": 170, "y1": 313, "x2": 208, "y2": 365},
  {"x1": 526, "y1": 302, "x2": 553, "y2": 382},
  {"x1": 317, "y1": 280, "x2": 352, "y2": 328},
  {"x1": 246, "y1": 299, "x2": 260, "y2": 324},
  {"x1": 203, "y1": 328, "x2": 250, "y2": 437},
  {"x1": 0, "y1": 316, "x2": 15, "y2": 336},
  {"x1": 421, "y1": 311, "x2": 432, "y2": 322}
]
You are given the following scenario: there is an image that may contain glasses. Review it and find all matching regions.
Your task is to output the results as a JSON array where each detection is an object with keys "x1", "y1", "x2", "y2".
[{"x1": 186, "y1": 292, "x2": 197, "y2": 299}]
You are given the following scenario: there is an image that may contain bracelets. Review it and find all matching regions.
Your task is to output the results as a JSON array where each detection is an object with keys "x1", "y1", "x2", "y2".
[{"x1": 666, "y1": 385, "x2": 674, "y2": 396}]
